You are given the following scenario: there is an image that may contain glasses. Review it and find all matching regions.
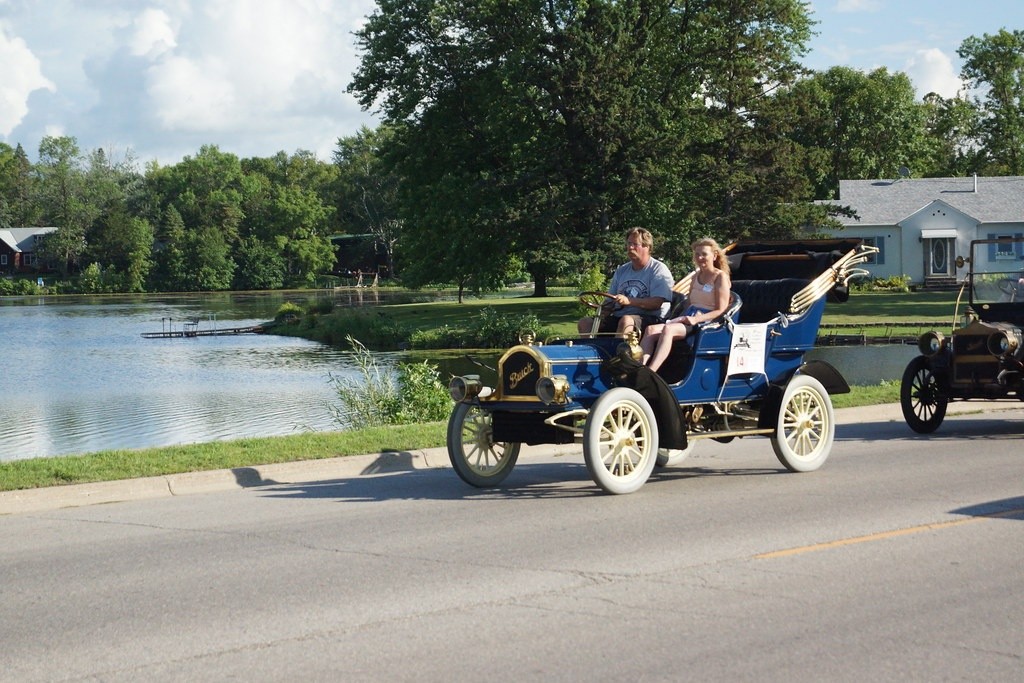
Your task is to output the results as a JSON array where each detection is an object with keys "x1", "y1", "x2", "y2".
[{"x1": 626, "y1": 240, "x2": 640, "y2": 247}]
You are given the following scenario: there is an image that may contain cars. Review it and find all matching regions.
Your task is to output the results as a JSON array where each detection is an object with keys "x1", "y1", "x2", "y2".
[
  {"x1": 899, "y1": 237, "x2": 1024, "y2": 436},
  {"x1": 446, "y1": 239, "x2": 880, "y2": 496}
]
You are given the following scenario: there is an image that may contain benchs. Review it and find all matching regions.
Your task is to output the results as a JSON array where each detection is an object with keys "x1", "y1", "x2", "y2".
[{"x1": 731, "y1": 278, "x2": 815, "y2": 323}]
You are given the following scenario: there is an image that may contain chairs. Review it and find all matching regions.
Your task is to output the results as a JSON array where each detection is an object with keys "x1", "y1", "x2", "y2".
[{"x1": 662, "y1": 289, "x2": 743, "y2": 382}]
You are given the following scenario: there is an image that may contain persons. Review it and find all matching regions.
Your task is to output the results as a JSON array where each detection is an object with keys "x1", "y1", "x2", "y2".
[
  {"x1": 577, "y1": 226, "x2": 674, "y2": 339},
  {"x1": 639, "y1": 238, "x2": 732, "y2": 373}
]
